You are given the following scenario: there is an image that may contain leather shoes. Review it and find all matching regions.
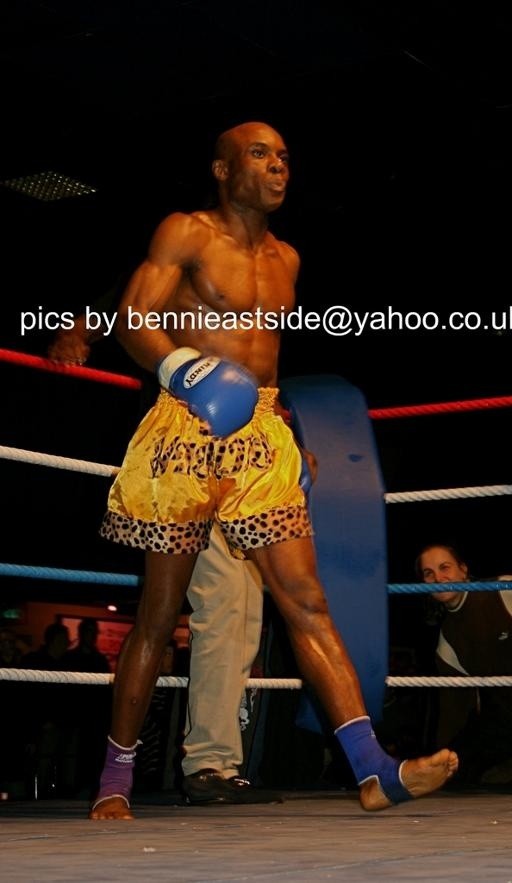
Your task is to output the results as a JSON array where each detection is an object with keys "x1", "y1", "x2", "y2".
[{"x1": 181, "y1": 773, "x2": 280, "y2": 806}]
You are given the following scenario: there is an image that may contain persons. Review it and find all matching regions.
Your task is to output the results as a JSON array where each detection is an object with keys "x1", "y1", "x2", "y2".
[
  {"x1": 180, "y1": 398, "x2": 321, "y2": 807},
  {"x1": 87, "y1": 119, "x2": 461, "y2": 823},
  {"x1": 0, "y1": 543, "x2": 511, "y2": 804}
]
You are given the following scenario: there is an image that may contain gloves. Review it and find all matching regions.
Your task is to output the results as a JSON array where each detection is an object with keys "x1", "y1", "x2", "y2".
[{"x1": 157, "y1": 345, "x2": 259, "y2": 438}]
